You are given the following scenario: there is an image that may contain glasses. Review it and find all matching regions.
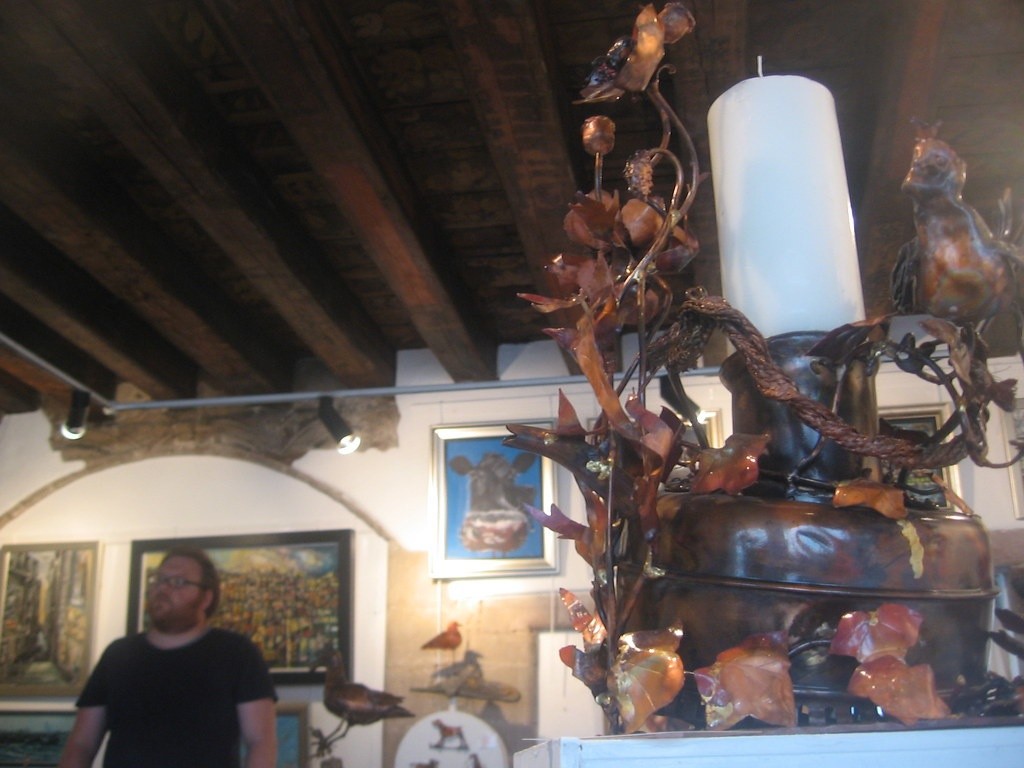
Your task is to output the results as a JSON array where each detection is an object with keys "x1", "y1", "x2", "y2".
[{"x1": 145, "y1": 568, "x2": 206, "y2": 591}]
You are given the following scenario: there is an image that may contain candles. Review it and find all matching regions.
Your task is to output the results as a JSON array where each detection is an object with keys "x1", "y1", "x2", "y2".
[{"x1": 707, "y1": 54, "x2": 867, "y2": 354}]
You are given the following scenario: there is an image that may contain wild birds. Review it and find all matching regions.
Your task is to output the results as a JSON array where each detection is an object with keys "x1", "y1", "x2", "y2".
[
  {"x1": 420, "y1": 619, "x2": 482, "y2": 682},
  {"x1": 308, "y1": 644, "x2": 414, "y2": 754}
]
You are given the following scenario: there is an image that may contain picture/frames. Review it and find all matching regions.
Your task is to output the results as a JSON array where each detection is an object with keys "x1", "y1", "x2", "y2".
[
  {"x1": 429, "y1": 420, "x2": 561, "y2": 576},
  {"x1": 0, "y1": 540, "x2": 107, "y2": 697},
  {"x1": 586, "y1": 408, "x2": 724, "y2": 464},
  {"x1": 127, "y1": 528, "x2": 356, "y2": 686},
  {"x1": 875, "y1": 402, "x2": 962, "y2": 515},
  {"x1": 238, "y1": 701, "x2": 309, "y2": 768},
  {"x1": 1002, "y1": 397, "x2": 1024, "y2": 519}
]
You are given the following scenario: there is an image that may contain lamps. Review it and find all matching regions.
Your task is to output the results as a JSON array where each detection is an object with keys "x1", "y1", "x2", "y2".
[
  {"x1": 60, "y1": 389, "x2": 88, "y2": 440},
  {"x1": 317, "y1": 396, "x2": 361, "y2": 454}
]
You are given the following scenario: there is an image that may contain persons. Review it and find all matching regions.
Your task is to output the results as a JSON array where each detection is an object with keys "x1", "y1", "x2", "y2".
[{"x1": 55, "y1": 544, "x2": 279, "y2": 768}]
